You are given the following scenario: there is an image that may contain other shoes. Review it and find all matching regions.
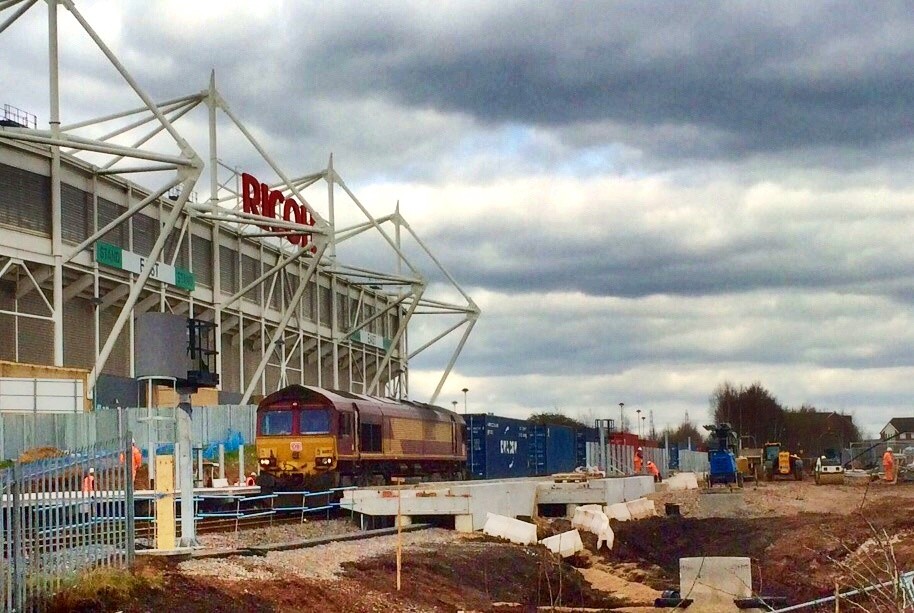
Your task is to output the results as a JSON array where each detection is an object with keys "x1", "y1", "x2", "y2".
[{"x1": 887, "y1": 480, "x2": 893, "y2": 483}]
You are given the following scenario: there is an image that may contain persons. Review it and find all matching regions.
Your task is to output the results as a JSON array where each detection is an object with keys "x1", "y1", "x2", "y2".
[
  {"x1": 645, "y1": 460, "x2": 662, "y2": 483},
  {"x1": 883, "y1": 447, "x2": 894, "y2": 482},
  {"x1": 246, "y1": 472, "x2": 257, "y2": 486},
  {"x1": 120, "y1": 437, "x2": 141, "y2": 492},
  {"x1": 84, "y1": 467, "x2": 100, "y2": 492},
  {"x1": 634, "y1": 446, "x2": 643, "y2": 474}
]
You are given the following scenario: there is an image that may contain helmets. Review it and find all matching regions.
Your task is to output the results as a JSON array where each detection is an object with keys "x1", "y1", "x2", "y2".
[
  {"x1": 250, "y1": 472, "x2": 256, "y2": 476},
  {"x1": 886, "y1": 447, "x2": 893, "y2": 452},
  {"x1": 646, "y1": 461, "x2": 651, "y2": 465},
  {"x1": 638, "y1": 446, "x2": 643, "y2": 450},
  {"x1": 89, "y1": 468, "x2": 94, "y2": 473}
]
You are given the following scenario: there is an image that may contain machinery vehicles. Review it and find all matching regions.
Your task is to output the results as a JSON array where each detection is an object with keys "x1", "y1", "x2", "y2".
[
  {"x1": 814, "y1": 456, "x2": 843, "y2": 484},
  {"x1": 761, "y1": 441, "x2": 804, "y2": 484}
]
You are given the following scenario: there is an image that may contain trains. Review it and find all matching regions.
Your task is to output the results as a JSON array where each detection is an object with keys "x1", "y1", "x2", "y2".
[{"x1": 252, "y1": 383, "x2": 470, "y2": 501}]
